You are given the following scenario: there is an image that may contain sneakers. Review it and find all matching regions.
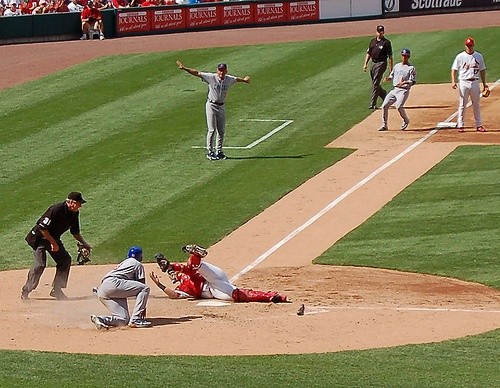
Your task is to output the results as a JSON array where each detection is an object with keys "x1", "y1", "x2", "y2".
[
  {"x1": 458, "y1": 126, "x2": 464, "y2": 132},
  {"x1": 216, "y1": 152, "x2": 228, "y2": 159},
  {"x1": 477, "y1": 126, "x2": 487, "y2": 132},
  {"x1": 90, "y1": 315, "x2": 113, "y2": 329},
  {"x1": 128, "y1": 319, "x2": 151, "y2": 327},
  {"x1": 186, "y1": 244, "x2": 208, "y2": 258},
  {"x1": 378, "y1": 126, "x2": 388, "y2": 131},
  {"x1": 207, "y1": 152, "x2": 219, "y2": 160},
  {"x1": 268, "y1": 291, "x2": 286, "y2": 302},
  {"x1": 80, "y1": 36, "x2": 87, "y2": 39},
  {"x1": 50, "y1": 289, "x2": 68, "y2": 300},
  {"x1": 100, "y1": 36, "x2": 104, "y2": 40},
  {"x1": 401, "y1": 119, "x2": 410, "y2": 130},
  {"x1": 369, "y1": 105, "x2": 377, "y2": 109},
  {"x1": 21, "y1": 292, "x2": 28, "y2": 299}
]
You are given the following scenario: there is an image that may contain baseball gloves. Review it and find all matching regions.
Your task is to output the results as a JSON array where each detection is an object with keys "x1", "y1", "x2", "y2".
[
  {"x1": 481, "y1": 85, "x2": 490, "y2": 97},
  {"x1": 76, "y1": 243, "x2": 92, "y2": 265},
  {"x1": 154, "y1": 252, "x2": 169, "y2": 272}
]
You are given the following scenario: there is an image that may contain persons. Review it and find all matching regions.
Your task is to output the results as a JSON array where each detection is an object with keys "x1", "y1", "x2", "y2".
[
  {"x1": 149, "y1": 244, "x2": 287, "y2": 300},
  {"x1": 4, "y1": 3, "x2": 22, "y2": 16},
  {"x1": 35, "y1": 0, "x2": 175, "y2": 13},
  {"x1": 79, "y1": 0, "x2": 105, "y2": 40},
  {"x1": 0, "y1": 2, "x2": 5, "y2": 17},
  {"x1": 363, "y1": 25, "x2": 393, "y2": 110},
  {"x1": 175, "y1": 59, "x2": 251, "y2": 160},
  {"x1": 451, "y1": 37, "x2": 488, "y2": 132},
  {"x1": 90, "y1": 246, "x2": 152, "y2": 331},
  {"x1": 21, "y1": 191, "x2": 93, "y2": 299},
  {"x1": 378, "y1": 47, "x2": 416, "y2": 130}
]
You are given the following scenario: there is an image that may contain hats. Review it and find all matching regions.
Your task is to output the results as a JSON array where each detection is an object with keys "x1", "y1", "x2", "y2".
[
  {"x1": 465, "y1": 38, "x2": 475, "y2": 45},
  {"x1": 401, "y1": 48, "x2": 411, "y2": 55},
  {"x1": 128, "y1": 246, "x2": 142, "y2": 257},
  {"x1": 377, "y1": 26, "x2": 385, "y2": 32},
  {"x1": 11, "y1": 0, "x2": 18, "y2": 5},
  {"x1": 218, "y1": 64, "x2": 227, "y2": 69},
  {"x1": 88, "y1": 1, "x2": 93, "y2": 5},
  {"x1": 68, "y1": 192, "x2": 86, "y2": 203},
  {"x1": 39, "y1": 0, "x2": 45, "y2": 4}
]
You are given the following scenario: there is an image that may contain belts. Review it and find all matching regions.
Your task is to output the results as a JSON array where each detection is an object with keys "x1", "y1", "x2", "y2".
[
  {"x1": 467, "y1": 78, "x2": 478, "y2": 80},
  {"x1": 372, "y1": 58, "x2": 386, "y2": 62},
  {"x1": 210, "y1": 100, "x2": 224, "y2": 105}
]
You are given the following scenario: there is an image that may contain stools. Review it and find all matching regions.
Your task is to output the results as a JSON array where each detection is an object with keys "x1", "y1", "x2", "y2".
[{"x1": 87, "y1": 27, "x2": 99, "y2": 39}]
